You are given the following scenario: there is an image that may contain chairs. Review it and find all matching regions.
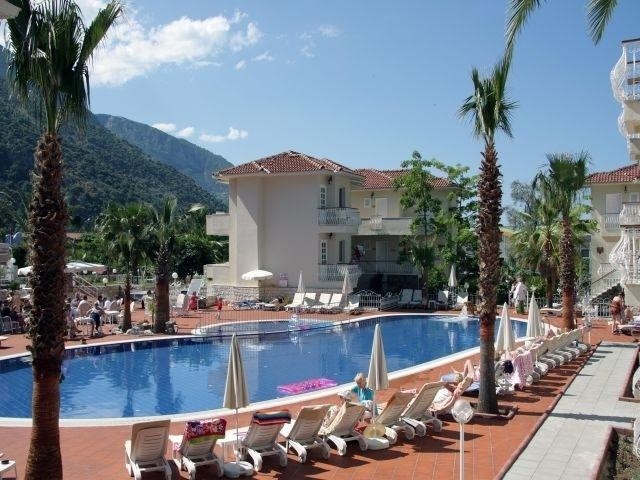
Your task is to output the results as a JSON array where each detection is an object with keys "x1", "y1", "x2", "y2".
[
  {"x1": 143, "y1": 292, "x2": 217, "y2": 317},
  {"x1": 277, "y1": 404, "x2": 331, "y2": 464},
  {"x1": 227, "y1": 409, "x2": 292, "y2": 471},
  {"x1": 319, "y1": 326, "x2": 592, "y2": 458},
  {"x1": 168, "y1": 417, "x2": 227, "y2": 480},
  {"x1": 395, "y1": 288, "x2": 468, "y2": 310},
  {"x1": 233, "y1": 290, "x2": 366, "y2": 314},
  {"x1": 124, "y1": 419, "x2": 172, "y2": 480},
  {"x1": 538, "y1": 298, "x2": 640, "y2": 337},
  {"x1": 0, "y1": 298, "x2": 135, "y2": 337}
]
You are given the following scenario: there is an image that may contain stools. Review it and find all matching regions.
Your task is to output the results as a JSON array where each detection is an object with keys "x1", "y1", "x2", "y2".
[{"x1": 0, "y1": 458, "x2": 18, "y2": 480}]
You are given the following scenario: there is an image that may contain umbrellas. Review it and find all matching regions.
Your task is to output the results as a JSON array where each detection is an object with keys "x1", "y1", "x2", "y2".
[
  {"x1": 218, "y1": 329, "x2": 258, "y2": 480},
  {"x1": 523, "y1": 291, "x2": 549, "y2": 378},
  {"x1": 297, "y1": 269, "x2": 306, "y2": 294},
  {"x1": 18, "y1": 261, "x2": 110, "y2": 278},
  {"x1": 447, "y1": 318, "x2": 458, "y2": 354},
  {"x1": 341, "y1": 267, "x2": 353, "y2": 314},
  {"x1": 362, "y1": 323, "x2": 389, "y2": 451},
  {"x1": 494, "y1": 300, "x2": 515, "y2": 396},
  {"x1": 240, "y1": 268, "x2": 275, "y2": 281},
  {"x1": 446, "y1": 264, "x2": 458, "y2": 309}
]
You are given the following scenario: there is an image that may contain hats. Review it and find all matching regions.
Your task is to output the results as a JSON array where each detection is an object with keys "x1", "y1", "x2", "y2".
[{"x1": 337, "y1": 391, "x2": 352, "y2": 400}]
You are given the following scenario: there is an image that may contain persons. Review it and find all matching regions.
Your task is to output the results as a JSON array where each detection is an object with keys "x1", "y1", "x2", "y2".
[
  {"x1": 610, "y1": 291, "x2": 624, "y2": 335},
  {"x1": 531, "y1": 329, "x2": 556, "y2": 345},
  {"x1": 624, "y1": 304, "x2": 634, "y2": 323},
  {"x1": 341, "y1": 371, "x2": 379, "y2": 420},
  {"x1": 510, "y1": 276, "x2": 528, "y2": 316},
  {"x1": 1, "y1": 277, "x2": 284, "y2": 344},
  {"x1": 450, "y1": 358, "x2": 514, "y2": 382}
]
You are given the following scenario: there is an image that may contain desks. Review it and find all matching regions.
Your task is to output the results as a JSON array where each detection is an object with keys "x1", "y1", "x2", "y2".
[{"x1": 215, "y1": 431, "x2": 241, "y2": 463}]
